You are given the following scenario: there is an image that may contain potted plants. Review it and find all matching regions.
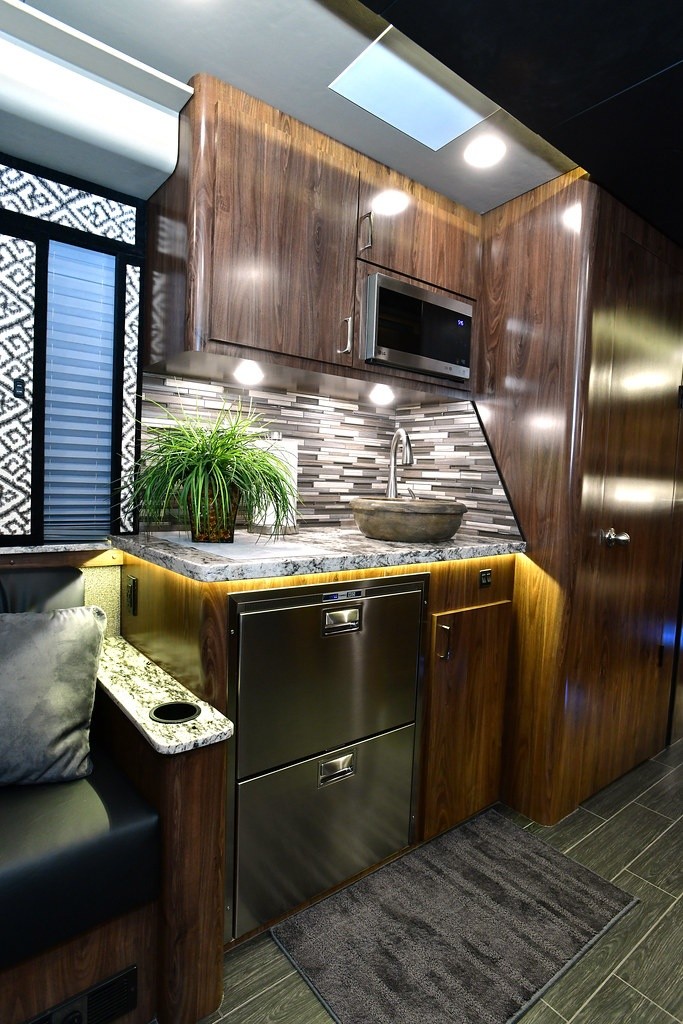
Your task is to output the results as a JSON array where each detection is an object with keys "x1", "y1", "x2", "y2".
[{"x1": 118, "y1": 382, "x2": 305, "y2": 547}]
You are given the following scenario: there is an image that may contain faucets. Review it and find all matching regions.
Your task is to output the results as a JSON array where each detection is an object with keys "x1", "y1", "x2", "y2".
[{"x1": 385, "y1": 428, "x2": 412, "y2": 498}]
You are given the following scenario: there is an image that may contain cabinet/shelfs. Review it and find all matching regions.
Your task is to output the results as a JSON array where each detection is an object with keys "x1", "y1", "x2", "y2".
[
  {"x1": 482, "y1": 167, "x2": 683, "y2": 825},
  {"x1": 139, "y1": 73, "x2": 481, "y2": 408},
  {"x1": 422, "y1": 599, "x2": 512, "y2": 840}
]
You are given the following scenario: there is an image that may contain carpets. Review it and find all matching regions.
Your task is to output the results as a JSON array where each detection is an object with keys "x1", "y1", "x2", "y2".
[{"x1": 271, "y1": 809, "x2": 641, "y2": 1024}]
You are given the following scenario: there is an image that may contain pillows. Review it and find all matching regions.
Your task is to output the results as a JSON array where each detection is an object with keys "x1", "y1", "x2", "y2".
[{"x1": 0, "y1": 606, "x2": 106, "y2": 783}]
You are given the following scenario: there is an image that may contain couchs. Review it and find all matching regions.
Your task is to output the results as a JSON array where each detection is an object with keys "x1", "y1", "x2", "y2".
[{"x1": 0, "y1": 542, "x2": 235, "y2": 1024}]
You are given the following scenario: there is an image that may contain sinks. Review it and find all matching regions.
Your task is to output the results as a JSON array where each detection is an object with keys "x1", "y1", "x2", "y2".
[{"x1": 351, "y1": 496, "x2": 467, "y2": 544}]
